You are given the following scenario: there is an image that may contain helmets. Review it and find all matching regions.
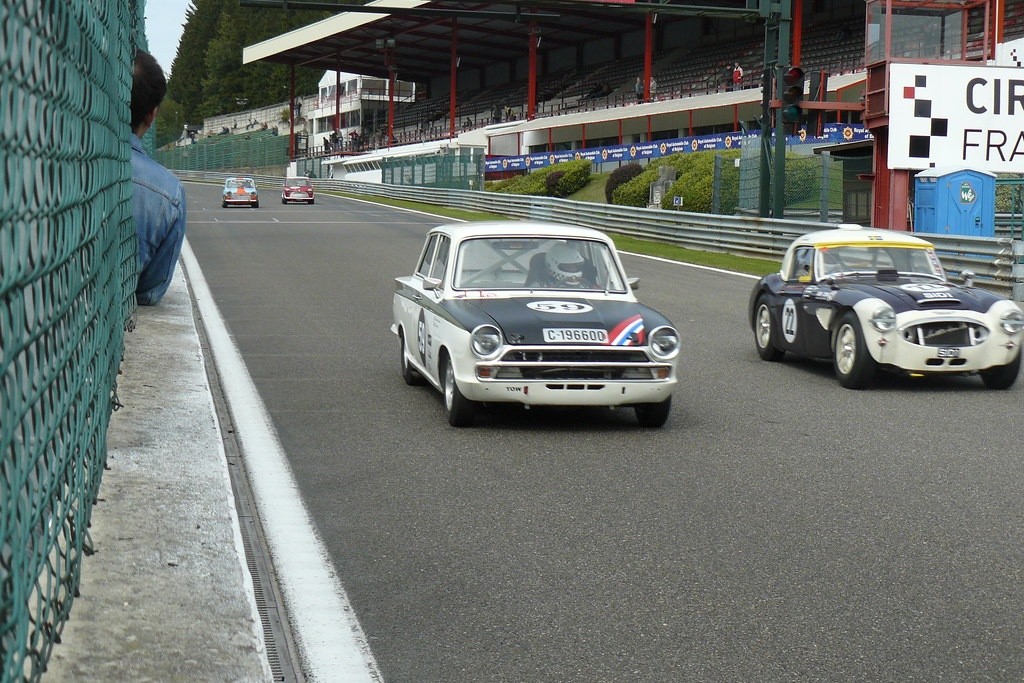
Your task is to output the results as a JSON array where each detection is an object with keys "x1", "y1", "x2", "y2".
[{"x1": 544, "y1": 243, "x2": 584, "y2": 286}]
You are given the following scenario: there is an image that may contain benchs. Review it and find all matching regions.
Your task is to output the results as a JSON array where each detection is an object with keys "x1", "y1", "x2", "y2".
[{"x1": 374, "y1": 0, "x2": 1024, "y2": 133}]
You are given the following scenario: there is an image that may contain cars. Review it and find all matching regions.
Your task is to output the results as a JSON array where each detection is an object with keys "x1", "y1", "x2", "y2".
[
  {"x1": 390, "y1": 218, "x2": 683, "y2": 429},
  {"x1": 220, "y1": 177, "x2": 260, "y2": 209},
  {"x1": 746, "y1": 224, "x2": 1023, "y2": 392},
  {"x1": 281, "y1": 176, "x2": 315, "y2": 204}
]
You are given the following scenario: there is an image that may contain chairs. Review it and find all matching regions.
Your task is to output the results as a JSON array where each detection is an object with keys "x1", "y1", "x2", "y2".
[{"x1": 526, "y1": 253, "x2": 600, "y2": 290}]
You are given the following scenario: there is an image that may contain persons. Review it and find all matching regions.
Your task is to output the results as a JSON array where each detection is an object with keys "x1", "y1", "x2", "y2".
[
  {"x1": 531, "y1": 241, "x2": 601, "y2": 289},
  {"x1": 798, "y1": 249, "x2": 841, "y2": 278},
  {"x1": 733, "y1": 62, "x2": 743, "y2": 92},
  {"x1": 130, "y1": 47, "x2": 186, "y2": 307},
  {"x1": 636, "y1": 77, "x2": 643, "y2": 104},
  {"x1": 493, "y1": 104, "x2": 502, "y2": 123},
  {"x1": 505, "y1": 105, "x2": 516, "y2": 121},
  {"x1": 650, "y1": 77, "x2": 657, "y2": 102},
  {"x1": 322, "y1": 125, "x2": 397, "y2": 155},
  {"x1": 190, "y1": 117, "x2": 278, "y2": 144},
  {"x1": 725, "y1": 64, "x2": 734, "y2": 92},
  {"x1": 466, "y1": 117, "x2": 472, "y2": 132}
]
